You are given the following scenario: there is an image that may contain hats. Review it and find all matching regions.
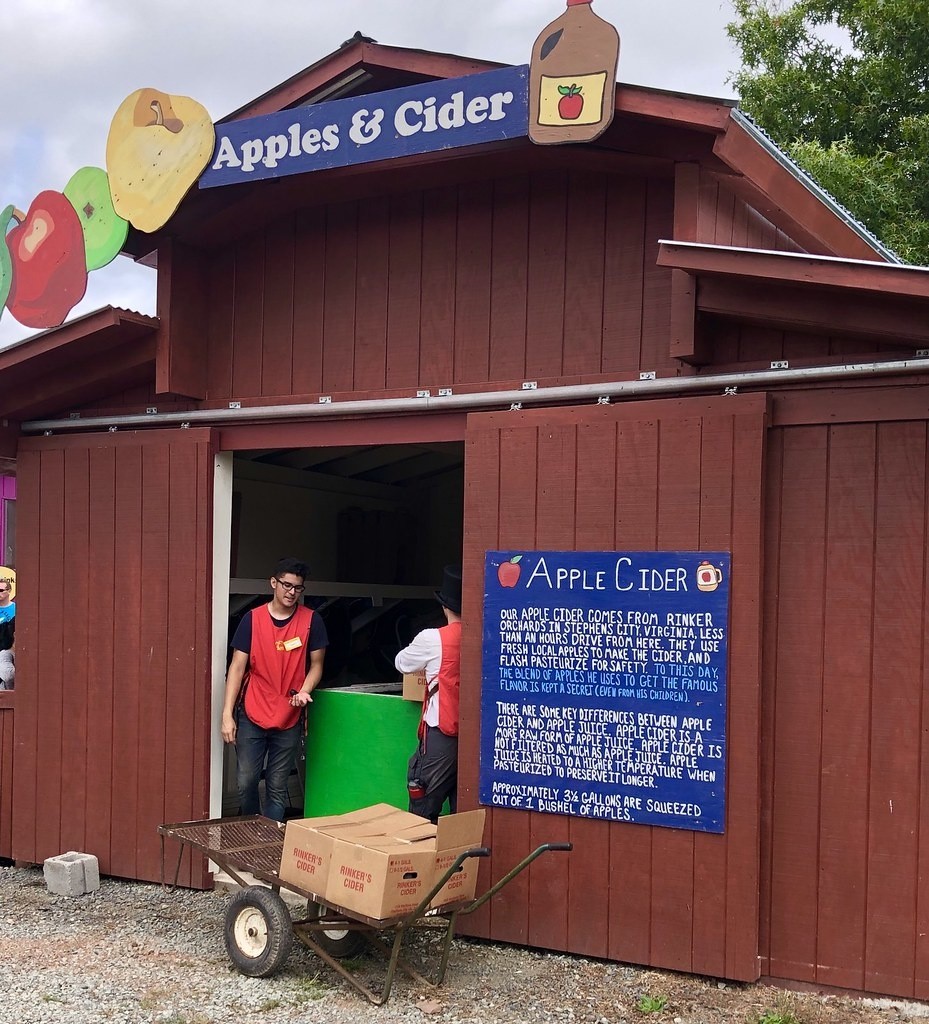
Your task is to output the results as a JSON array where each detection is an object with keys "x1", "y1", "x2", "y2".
[{"x1": 433, "y1": 566, "x2": 462, "y2": 613}]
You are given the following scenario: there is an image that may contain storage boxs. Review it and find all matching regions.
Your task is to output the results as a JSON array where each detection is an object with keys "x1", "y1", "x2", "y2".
[
  {"x1": 277, "y1": 803, "x2": 486, "y2": 919},
  {"x1": 403, "y1": 669, "x2": 428, "y2": 702}
]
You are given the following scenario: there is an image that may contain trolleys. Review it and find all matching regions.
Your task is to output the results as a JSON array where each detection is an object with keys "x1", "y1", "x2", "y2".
[{"x1": 158, "y1": 813, "x2": 599, "y2": 1008}]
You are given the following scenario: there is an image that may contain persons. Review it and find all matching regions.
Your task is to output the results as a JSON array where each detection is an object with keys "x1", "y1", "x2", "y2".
[
  {"x1": 0, "y1": 581, "x2": 15, "y2": 690},
  {"x1": 394, "y1": 565, "x2": 461, "y2": 823},
  {"x1": 220, "y1": 558, "x2": 329, "y2": 886}
]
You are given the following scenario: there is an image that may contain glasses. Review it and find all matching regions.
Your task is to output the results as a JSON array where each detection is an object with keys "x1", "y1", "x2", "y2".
[
  {"x1": 0, "y1": 589, "x2": 9, "y2": 592},
  {"x1": 274, "y1": 577, "x2": 306, "y2": 593}
]
